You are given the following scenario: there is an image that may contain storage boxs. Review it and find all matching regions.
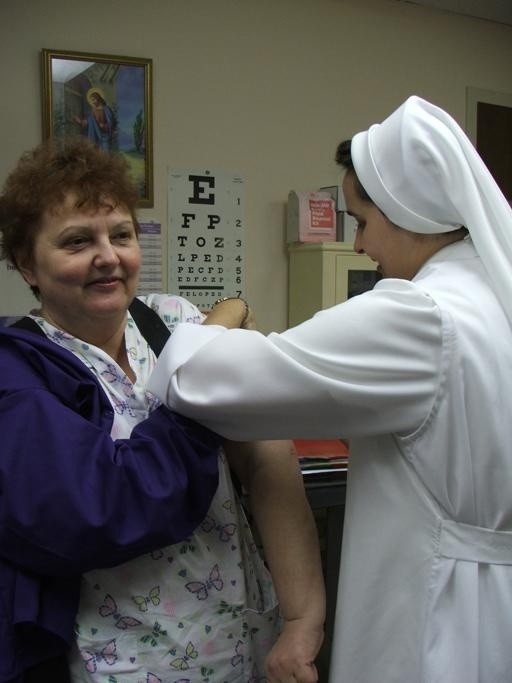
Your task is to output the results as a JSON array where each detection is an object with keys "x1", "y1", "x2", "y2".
[
  {"x1": 319, "y1": 184, "x2": 349, "y2": 215},
  {"x1": 335, "y1": 209, "x2": 361, "y2": 242}
]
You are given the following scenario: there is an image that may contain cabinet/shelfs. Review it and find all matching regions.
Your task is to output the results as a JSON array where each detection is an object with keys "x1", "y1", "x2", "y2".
[
  {"x1": 285, "y1": 238, "x2": 380, "y2": 334},
  {"x1": 236, "y1": 473, "x2": 345, "y2": 680}
]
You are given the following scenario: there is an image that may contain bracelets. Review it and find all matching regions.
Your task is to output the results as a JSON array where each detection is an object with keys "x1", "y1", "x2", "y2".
[{"x1": 212, "y1": 295, "x2": 250, "y2": 329}]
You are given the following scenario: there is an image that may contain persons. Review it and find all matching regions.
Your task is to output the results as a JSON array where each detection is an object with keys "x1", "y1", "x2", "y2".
[
  {"x1": 72, "y1": 89, "x2": 117, "y2": 153},
  {"x1": 161, "y1": 101, "x2": 512, "y2": 683},
  {"x1": 0, "y1": 141, "x2": 325, "y2": 683}
]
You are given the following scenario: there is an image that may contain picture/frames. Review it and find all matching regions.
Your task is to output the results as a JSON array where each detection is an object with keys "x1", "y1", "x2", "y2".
[{"x1": 36, "y1": 44, "x2": 156, "y2": 213}]
